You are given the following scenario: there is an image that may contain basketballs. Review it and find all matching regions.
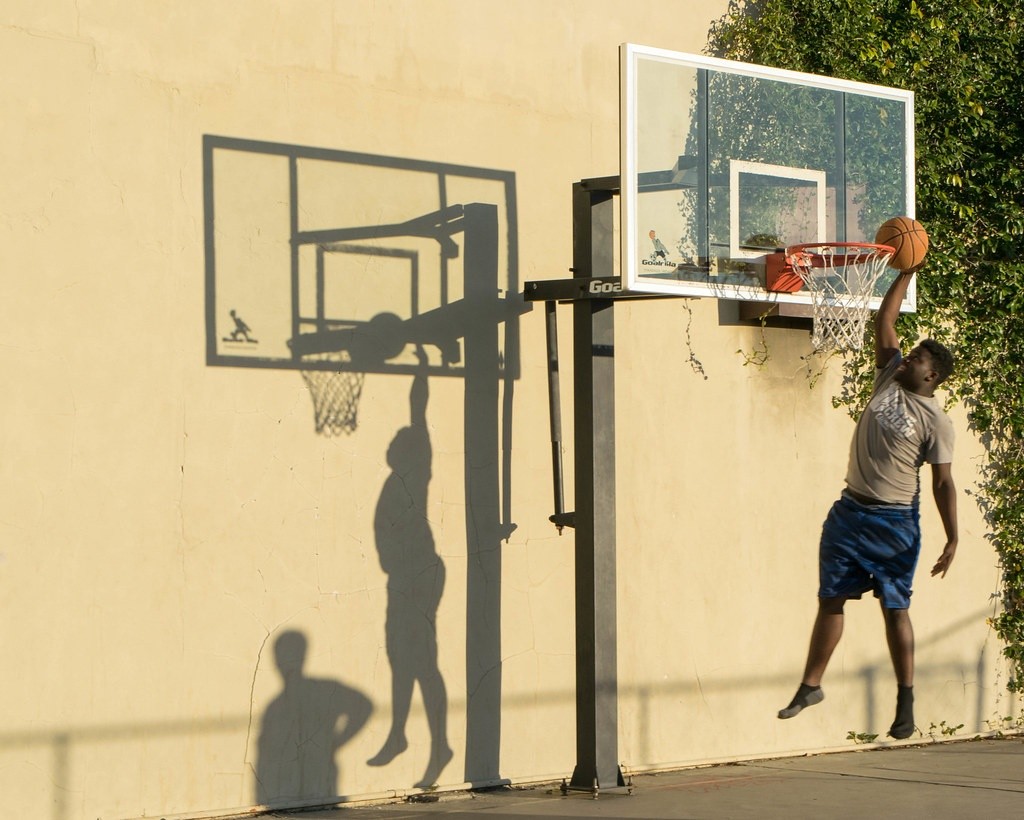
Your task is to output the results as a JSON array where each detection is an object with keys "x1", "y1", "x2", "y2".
[{"x1": 875, "y1": 215, "x2": 929, "y2": 270}]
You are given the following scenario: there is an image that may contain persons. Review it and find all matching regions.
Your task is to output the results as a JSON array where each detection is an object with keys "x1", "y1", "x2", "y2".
[{"x1": 776, "y1": 258, "x2": 959, "y2": 741}]
